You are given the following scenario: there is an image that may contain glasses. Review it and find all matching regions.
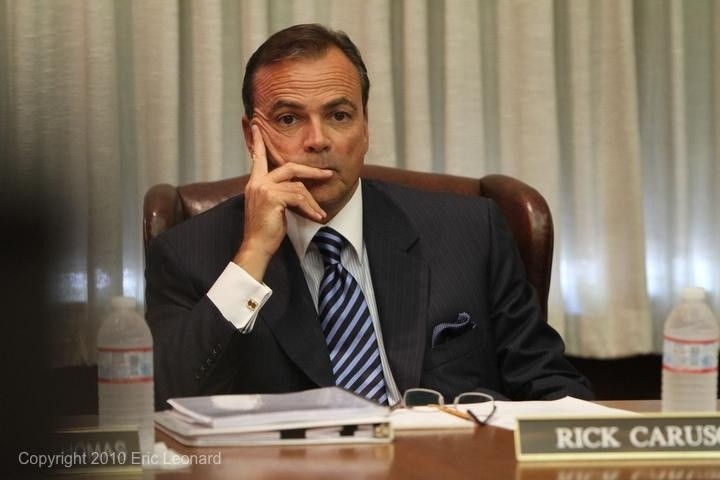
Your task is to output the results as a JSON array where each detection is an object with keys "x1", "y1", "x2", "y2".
[{"x1": 400, "y1": 387, "x2": 493, "y2": 425}]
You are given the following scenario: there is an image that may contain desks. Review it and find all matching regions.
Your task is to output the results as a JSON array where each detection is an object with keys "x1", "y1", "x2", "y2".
[{"x1": 0, "y1": 399, "x2": 720, "y2": 478}]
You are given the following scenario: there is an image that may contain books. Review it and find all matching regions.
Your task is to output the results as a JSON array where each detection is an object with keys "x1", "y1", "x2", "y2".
[
  {"x1": 163, "y1": 388, "x2": 391, "y2": 427},
  {"x1": 150, "y1": 386, "x2": 396, "y2": 446},
  {"x1": 180, "y1": 444, "x2": 395, "y2": 478},
  {"x1": 390, "y1": 404, "x2": 476, "y2": 432}
]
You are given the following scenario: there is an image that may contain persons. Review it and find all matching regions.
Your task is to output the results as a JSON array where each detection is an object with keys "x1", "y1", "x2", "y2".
[{"x1": 142, "y1": 22, "x2": 602, "y2": 401}]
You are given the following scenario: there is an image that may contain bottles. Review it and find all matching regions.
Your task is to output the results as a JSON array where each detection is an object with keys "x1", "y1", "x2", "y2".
[
  {"x1": 659, "y1": 286, "x2": 719, "y2": 414},
  {"x1": 95, "y1": 296, "x2": 157, "y2": 458}
]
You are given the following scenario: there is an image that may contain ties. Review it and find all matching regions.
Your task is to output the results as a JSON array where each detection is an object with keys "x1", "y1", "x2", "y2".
[{"x1": 312, "y1": 228, "x2": 389, "y2": 409}]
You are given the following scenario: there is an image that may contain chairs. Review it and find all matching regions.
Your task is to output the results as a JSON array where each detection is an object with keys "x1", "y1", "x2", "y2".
[{"x1": 139, "y1": 166, "x2": 555, "y2": 331}]
